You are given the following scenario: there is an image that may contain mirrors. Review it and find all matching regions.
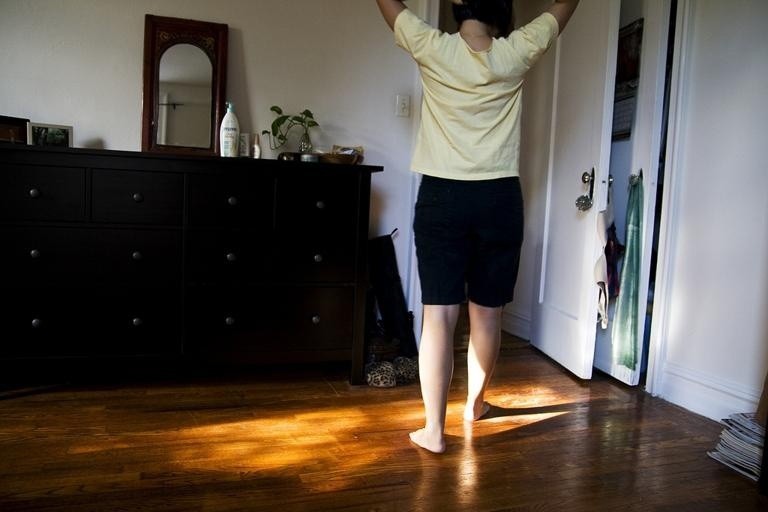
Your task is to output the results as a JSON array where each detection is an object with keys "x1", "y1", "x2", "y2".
[{"x1": 140, "y1": 13, "x2": 228, "y2": 156}]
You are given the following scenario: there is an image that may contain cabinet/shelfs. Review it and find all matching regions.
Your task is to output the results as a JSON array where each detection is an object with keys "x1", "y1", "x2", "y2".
[{"x1": 1, "y1": 145, "x2": 384, "y2": 392}]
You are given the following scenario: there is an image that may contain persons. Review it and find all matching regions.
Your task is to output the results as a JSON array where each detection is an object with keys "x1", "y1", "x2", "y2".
[{"x1": 376, "y1": 0, "x2": 580, "y2": 454}]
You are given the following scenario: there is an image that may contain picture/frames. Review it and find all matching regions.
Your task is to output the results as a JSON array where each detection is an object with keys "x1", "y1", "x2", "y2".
[
  {"x1": 0, "y1": 115, "x2": 30, "y2": 144},
  {"x1": 26, "y1": 121, "x2": 73, "y2": 149}
]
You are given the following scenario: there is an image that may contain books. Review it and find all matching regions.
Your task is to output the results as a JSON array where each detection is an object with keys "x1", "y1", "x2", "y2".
[{"x1": 706, "y1": 411, "x2": 766, "y2": 482}]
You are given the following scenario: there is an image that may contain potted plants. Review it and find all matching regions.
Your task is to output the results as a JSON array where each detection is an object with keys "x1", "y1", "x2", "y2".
[{"x1": 262, "y1": 105, "x2": 319, "y2": 152}]
